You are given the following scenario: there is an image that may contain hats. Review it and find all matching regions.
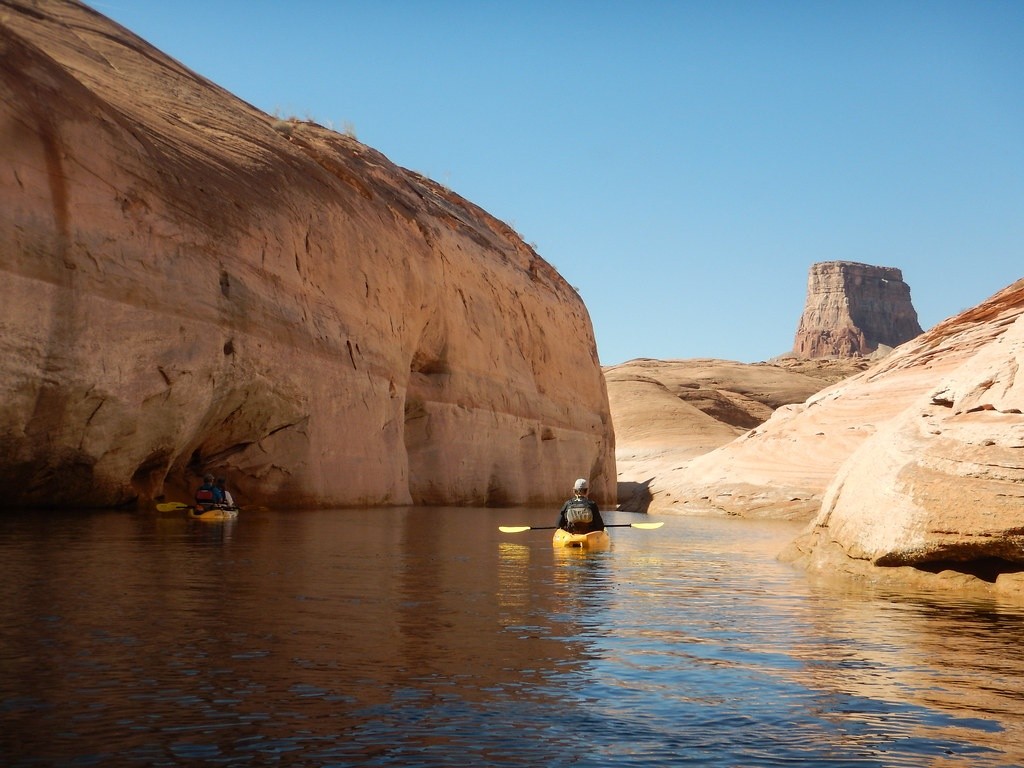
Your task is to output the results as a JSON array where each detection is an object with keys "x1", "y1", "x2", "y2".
[
  {"x1": 574, "y1": 478, "x2": 589, "y2": 489},
  {"x1": 203, "y1": 474, "x2": 215, "y2": 481}
]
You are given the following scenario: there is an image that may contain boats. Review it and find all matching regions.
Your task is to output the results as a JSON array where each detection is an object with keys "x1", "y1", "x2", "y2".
[
  {"x1": 552, "y1": 526, "x2": 612, "y2": 554},
  {"x1": 187, "y1": 507, "x2": 240, "y2": 521}
]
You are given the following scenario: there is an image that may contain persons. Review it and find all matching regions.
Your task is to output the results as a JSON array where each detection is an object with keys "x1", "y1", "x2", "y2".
[
  {"x1": 555, "y1": 478, "x2": 604, "y2": 535},
  {"x1": 195, "y1": 474, "x2": 234, "y2": 510}
]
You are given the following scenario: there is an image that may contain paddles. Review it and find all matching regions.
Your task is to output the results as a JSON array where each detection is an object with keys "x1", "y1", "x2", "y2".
[
  {"x1": 169, "y1": 502, "x2": 268, "y2": 511},
  {"x1": 156, "y1": 503, "x2": 265, "y2": 512},
  {"x1": 497, "y1": 522, "x2": 665, "y2": 534}
]
